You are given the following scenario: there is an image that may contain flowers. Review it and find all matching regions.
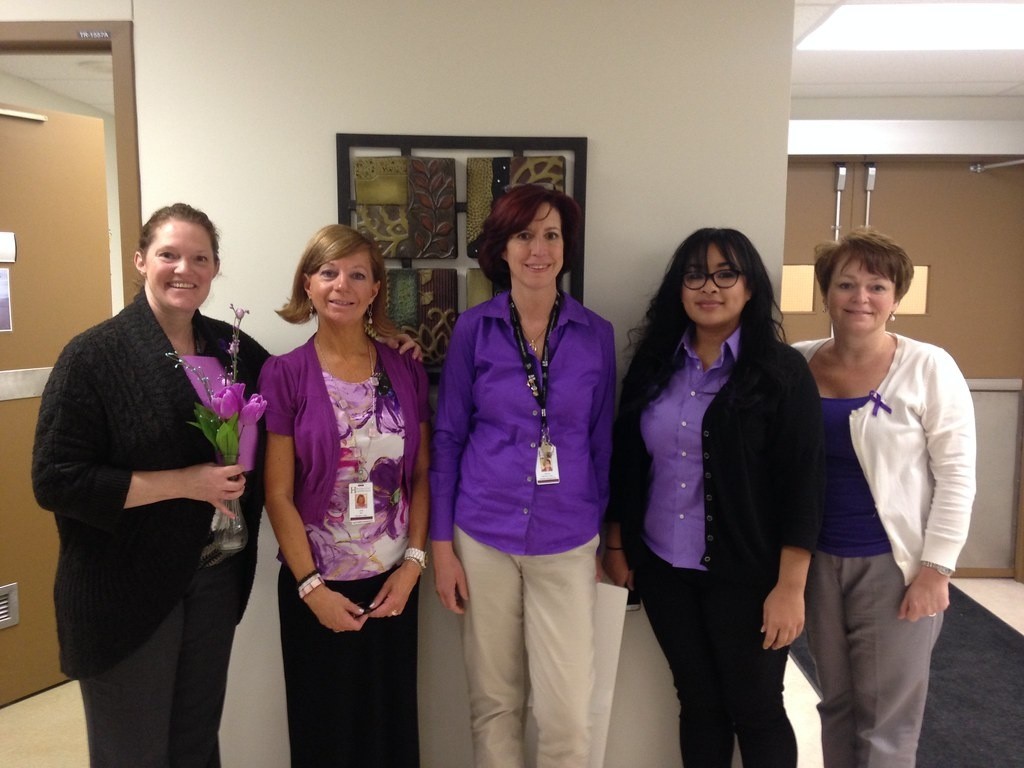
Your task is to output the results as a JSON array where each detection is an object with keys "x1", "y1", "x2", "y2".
[{"x1": 164, "y1": 302, "x2": 268, "y2": 454}]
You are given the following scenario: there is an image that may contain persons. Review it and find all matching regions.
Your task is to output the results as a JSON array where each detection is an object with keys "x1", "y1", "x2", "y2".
[
  {"x1": 789, "y1": 226, "x2": 979, "y2": 767},
  {"x1": 611, "y1": 224, "x2": 822, "y2": 768},
  {"x1": 429, "y1": 181, "x2": 621, "y2": 768},
  {"x1": 255, "y1": 223, "x2": 431, "y2": 765},
  {"x1": 25, "y1": 202, "x2": 426, "y2": 768}
]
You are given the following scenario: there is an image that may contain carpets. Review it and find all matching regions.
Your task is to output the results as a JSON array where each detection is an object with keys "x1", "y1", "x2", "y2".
[{"x1": 788, "y1": 582, "x2": 1024, "y2": 768}]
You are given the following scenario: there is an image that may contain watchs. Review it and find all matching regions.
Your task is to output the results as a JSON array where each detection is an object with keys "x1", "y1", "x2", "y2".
[
  {"x1": 921, "y1": 560, "x2": 954, "y2": 576},
  {"x1": 403, "y1": 547, "x2": 426, "y2": 569}
]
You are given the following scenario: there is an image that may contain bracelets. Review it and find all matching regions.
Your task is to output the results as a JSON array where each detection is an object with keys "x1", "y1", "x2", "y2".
[
  {"x1": 604, "y1": 545, "x2": 622, "y2": 551},
  {"x1": 404, "y1": 557, "x2": 424, "y2": 575},
  {"x1": 294, "y1": 568, "x2": 325, "y2": 599}
]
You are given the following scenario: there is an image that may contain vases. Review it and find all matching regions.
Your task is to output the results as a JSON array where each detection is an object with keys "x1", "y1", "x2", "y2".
[{"x1": 206, "y1": 449, "x2": 249, "y2": 554}]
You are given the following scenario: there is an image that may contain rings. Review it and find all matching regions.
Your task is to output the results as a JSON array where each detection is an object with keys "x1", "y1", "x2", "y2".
[{"x1": 928, "y1": 614, "x2": 937, "y2": 617}]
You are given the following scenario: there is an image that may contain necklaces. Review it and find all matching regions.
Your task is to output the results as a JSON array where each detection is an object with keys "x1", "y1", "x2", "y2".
[{"x1": 521, "y1": 321, "x2": 548, "y2": 354}]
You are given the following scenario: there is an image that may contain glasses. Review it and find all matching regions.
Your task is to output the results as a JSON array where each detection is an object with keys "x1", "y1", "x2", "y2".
[{"x1": 680, "y1": 269, "x2": 744, "y2": 290}]
